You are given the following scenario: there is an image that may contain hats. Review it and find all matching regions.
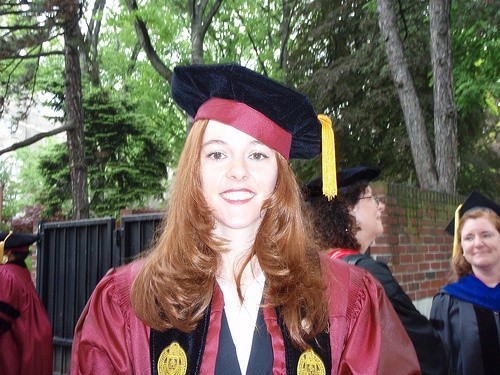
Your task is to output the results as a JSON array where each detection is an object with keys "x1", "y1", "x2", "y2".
[
  {"x1": 445, "y1": 192, "x2": 500, "y2": 264},
  {"x1": 0, "y1": 231, "x2": 41, "y2": 264},
  {"x1": 172, "y1": 63, "x2": 338, "y2": 200},
  {"x1": 302, "y1": 160, "x2": 382, "y2": 200}
]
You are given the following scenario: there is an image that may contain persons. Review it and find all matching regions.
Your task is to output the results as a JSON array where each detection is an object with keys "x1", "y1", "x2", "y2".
[
  {"x1": 429, "y1": 192, "x2": 500, "y2": 375},
  {"x1": 70, "y1": 64, "x2": 422, "y2": 375},
  {"x1": 304, "y1": 168, "x2": 446, "y2": 375},
  {"x1": 0, "y1": 231, "x2": 53, "y2": 375}
]
